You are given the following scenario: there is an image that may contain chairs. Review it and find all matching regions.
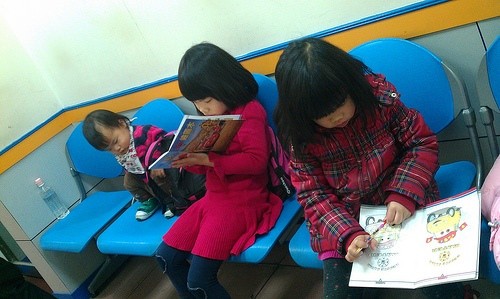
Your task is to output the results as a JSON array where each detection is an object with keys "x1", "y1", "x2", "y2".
[{"x1": 38, "y1": 36, "x2": 500, "y2": 295}]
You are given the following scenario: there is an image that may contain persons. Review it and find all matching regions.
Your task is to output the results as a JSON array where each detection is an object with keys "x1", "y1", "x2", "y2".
[
  {"x1": 479, "y1": 154, "x2": 500, "y2": 270},
  {"x1": 152, "y1": 43, "x2": 283, "y2": 299},
  {"x1": 273, "y1": 38, "x2": 441, "y2": 299},
  {"x1": 82, "y1": 109, "x2": 175, "y2": 221}
]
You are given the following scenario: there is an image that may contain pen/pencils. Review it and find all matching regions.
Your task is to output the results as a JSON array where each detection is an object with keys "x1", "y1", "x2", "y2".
[{"x1": 354, "y1": 219, "x2": 387, "y2": 254}]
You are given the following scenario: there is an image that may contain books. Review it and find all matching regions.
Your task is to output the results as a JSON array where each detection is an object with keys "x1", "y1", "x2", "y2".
[
  {"x1": 148, "y1": 115, "x2": 245, "y2": 170},
  {"x1": 347, "y1": 186, "x2": 481, "y2": 289}
]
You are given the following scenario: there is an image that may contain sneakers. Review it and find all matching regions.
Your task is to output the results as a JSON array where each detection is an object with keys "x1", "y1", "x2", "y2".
[
  {"x1": 164, "y1": 205, "x2": 174, "y2": 218},
  {"x1": 136, "y1": 197, "x2": 160, "y2": 221}
]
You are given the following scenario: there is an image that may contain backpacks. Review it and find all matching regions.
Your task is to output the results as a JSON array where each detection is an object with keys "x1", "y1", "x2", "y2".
[{"x1": 266, "y1": 126, "x2": 296, "y2": 203}]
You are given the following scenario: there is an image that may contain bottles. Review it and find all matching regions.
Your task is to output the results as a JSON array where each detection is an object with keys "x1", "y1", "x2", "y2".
[{"x1": 34, "y1": 177, "x2": 70, "y2": 220}]
